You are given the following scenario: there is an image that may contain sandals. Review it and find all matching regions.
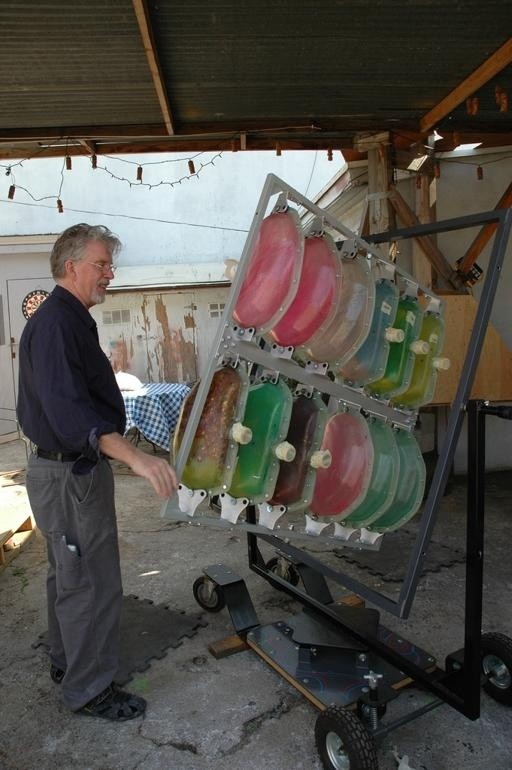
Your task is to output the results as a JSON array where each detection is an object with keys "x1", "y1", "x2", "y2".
[{"x1": 51, "y1": 664, "x2": 148, "y2": 721}]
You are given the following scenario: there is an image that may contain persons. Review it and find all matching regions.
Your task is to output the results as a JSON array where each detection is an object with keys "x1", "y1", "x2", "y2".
[{"x1": 15, "y1": 223, "x2": 181, "y2": 725}]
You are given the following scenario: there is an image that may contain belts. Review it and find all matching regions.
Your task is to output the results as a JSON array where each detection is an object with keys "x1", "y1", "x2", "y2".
[{"x1": 31, "y1": 446, "x2": 82, "y2": 462}]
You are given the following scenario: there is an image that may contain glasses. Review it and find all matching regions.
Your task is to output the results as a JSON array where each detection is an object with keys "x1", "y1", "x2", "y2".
[{"x1": 75, "y1": 257, "x2": 118, "y2": 272}]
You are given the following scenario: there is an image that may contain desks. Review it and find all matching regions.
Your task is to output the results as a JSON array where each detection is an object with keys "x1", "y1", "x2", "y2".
[{"x1": 121, "y1": 383, "x2": 191, "y2": 454}]
